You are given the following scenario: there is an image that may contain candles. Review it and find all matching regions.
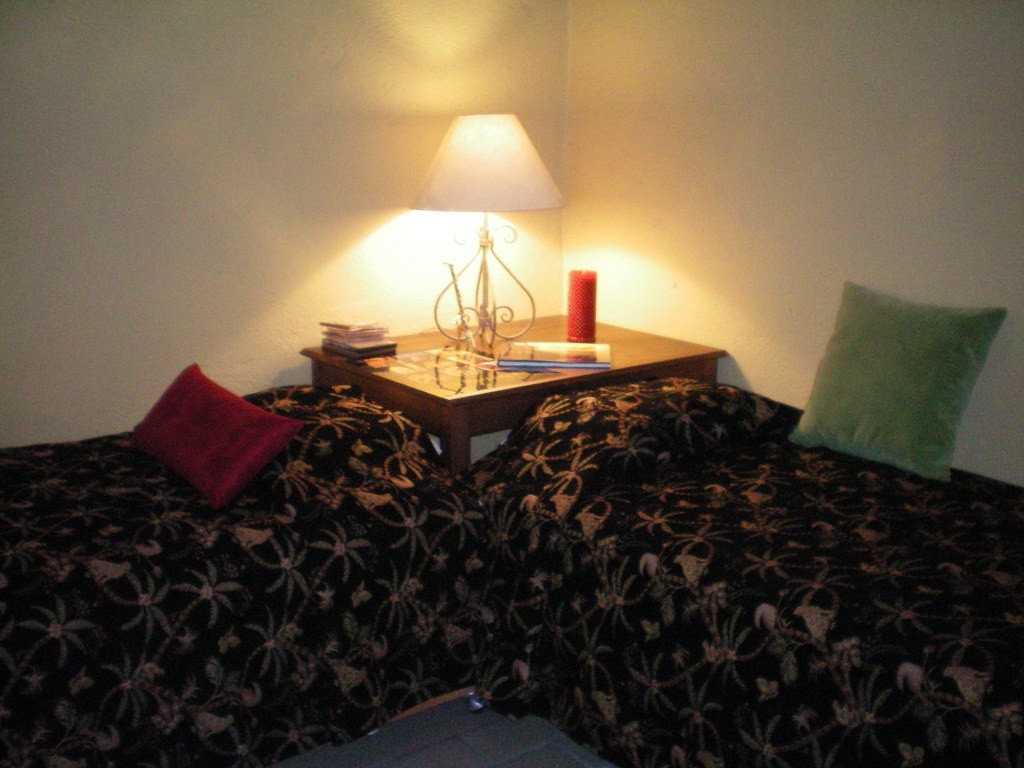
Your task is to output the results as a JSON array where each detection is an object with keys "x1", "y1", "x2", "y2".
[{"x1": 565, "y1": 266, "x2": 598, "y2": 342}]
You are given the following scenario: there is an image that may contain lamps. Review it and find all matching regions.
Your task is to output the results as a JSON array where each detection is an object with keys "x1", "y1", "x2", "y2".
[{"x1": 411, "y1": 114, "x2": 565, "y2": 348}]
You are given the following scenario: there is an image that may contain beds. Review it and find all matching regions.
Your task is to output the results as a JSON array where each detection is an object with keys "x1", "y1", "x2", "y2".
[
  {"x1": 459, "y1": 427, "x2": 1024, "y2": 768},
  {"x1": 0, "y1": 434, "x2": 480, "y2": 768}
]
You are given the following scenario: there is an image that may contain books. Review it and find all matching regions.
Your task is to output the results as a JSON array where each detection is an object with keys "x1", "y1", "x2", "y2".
[
  {"x1": 323, "y1": 335, "x2": 397, "y2": 359},
  {"x1": 497, "y1": 340, "x2": 613, "y2": 368}
]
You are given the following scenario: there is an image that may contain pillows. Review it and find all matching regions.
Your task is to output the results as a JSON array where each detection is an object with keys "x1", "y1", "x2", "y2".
[
  {"x1": 128, "y1": 364, "x2": 306, "y2": 515},
  {"x1": 787, "y1": 281, "x2": 1008, "y2": 486},
  {"x1": 234, "y1": 382, "x2": 444, "y2": 483},
  {"x1": 514, "y1": 376, "x2": 803, "y2": 479}
]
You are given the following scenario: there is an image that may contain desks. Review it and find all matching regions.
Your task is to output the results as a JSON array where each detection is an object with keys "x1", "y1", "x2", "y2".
[{"x1": 298, "y1": 314, "x2": 726, "y2": 478}]
